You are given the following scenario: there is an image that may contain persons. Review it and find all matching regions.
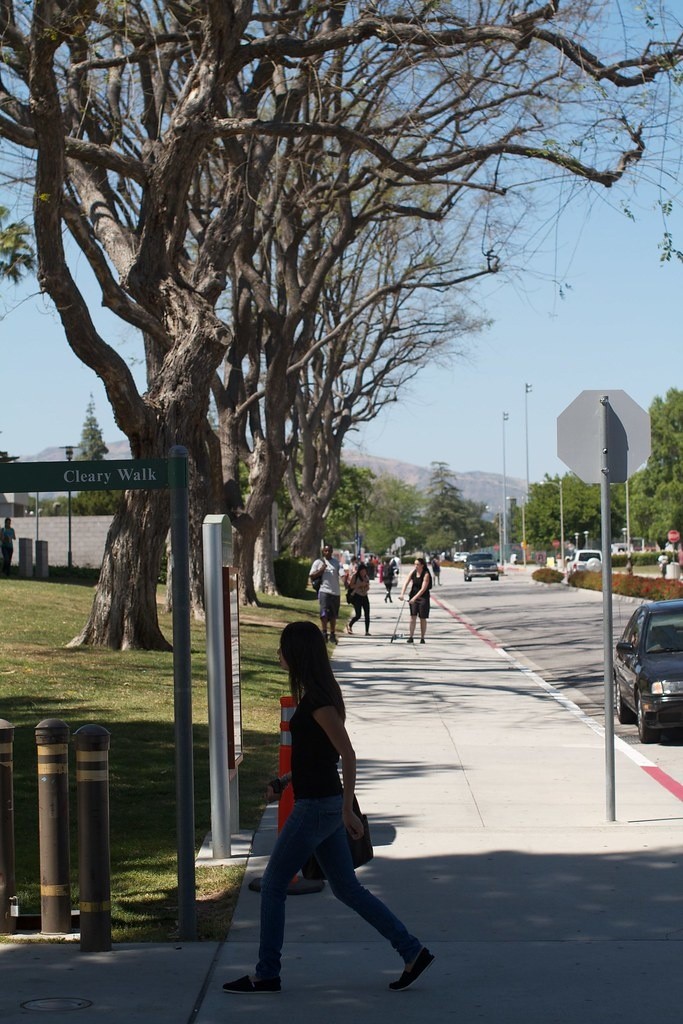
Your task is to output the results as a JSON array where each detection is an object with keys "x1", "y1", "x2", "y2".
[
  {"x1": 625, "y1": 550, "x2": 634, "y2": 575},
  {"x1": 310, "y1": 544, "x2": 349, "y2": 642},
  {"x1": 556, "y1": 553, "x2": 561, "y2": 559},
  {"x1": 432, "y1": 555, "x2": 441, "y2": 587},
  {"x1": 346, "y1": 564, "x2": 371, "y2": 636},
  {"x1": 333, "y1": 548, "x2": 403, "y2": 605},
  {"x1": 0, "y1": 518, "x2": 16, "y2": 576},
  {"x1": 538, "y1": 554, "x2": 545, "y2": 569},
  {"x1": 223, "y1": 621, "x2": 436, "y2": 993},
  {"x1": 658, "y1": 552, "x2": 668, "y2": 577},
  {"x1": 400, "y1": 558, "x2": 432, "y2": 644},
  {"x1": 678, "y1": 548, "x2": 683, "y2": 580}
]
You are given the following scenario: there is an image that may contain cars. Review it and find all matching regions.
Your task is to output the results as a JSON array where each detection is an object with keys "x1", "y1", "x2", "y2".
[
  {"x1": 612, "y1": 599, "x2": 683, "y2": 744},
  {"x1": 464, "y1": 554, "x2": 499, "y2": 582},
  {"x1": 454, "y1": 553, "x2": 470, "y2": 562}
]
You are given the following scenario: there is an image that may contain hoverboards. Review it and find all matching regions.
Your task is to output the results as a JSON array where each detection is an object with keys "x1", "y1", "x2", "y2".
[{"x1": 391, "y1": 598, "x2": 411, "y2": 643}]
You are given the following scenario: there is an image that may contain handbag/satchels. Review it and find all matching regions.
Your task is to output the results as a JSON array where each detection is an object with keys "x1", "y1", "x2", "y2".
[
  {"x1": 310, "y1": 569, "x2": 324, "y2": 591},
  {"x1": 346, "y1": 584, "x2": 355, "y2": 604},
  {"x1": 301, "y1": 794, "x2": 373, "y2": 880}
]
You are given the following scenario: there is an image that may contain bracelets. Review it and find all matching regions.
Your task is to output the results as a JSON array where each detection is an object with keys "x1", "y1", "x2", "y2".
[{"x1": 281, "y1": 774, "x2": 289, "y2": 787}]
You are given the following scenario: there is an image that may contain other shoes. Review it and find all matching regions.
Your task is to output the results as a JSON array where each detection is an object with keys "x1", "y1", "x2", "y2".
[
  {"x1": 388, "y1": 946, "x2": 436, "y2": 991},
  {"x1": 421, "y1": 639, "x2": 425, "y2": 643},
  {"x1": 322, "y1": 633, "x2": 338, "y2": 645},
  {"x1": 365, "y1": 633, "x2": 371, "y2": 636},
  {"x1": 347, "y1": 625, "x2": 353, "y2": 634},
  {"x1": 407, "y1": 637, "x2": 413, "y2": 643},
  {"x1": 223, "y1": 975, "x2": 282, "y2": 994}
]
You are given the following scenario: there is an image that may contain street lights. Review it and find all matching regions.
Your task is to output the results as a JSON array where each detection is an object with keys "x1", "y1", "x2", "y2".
[
  {"x1": 540, "y1": 481, "x2": 564, "y2": 571},
  {"x1": 503, "y1": 411, "x2": 509, "y2": 574},
  {"x1": 506, "y1": 496, "x2": 526, "y2": 567},
  {"x1": 525, "y1": 382, "x2": 532, "y2": 489},
  {"x1": 59, "y1": 445, "x2": 80, "y2": 570},
  {"x1": 584, "y1": 531, "x2": 589, "y2": 549},
  {"x1": 574, "y1": 533, "x2": 579, "y2": 549},
  {"x1": 621, "y1": 528, "x2": 627, "y2": 554}
]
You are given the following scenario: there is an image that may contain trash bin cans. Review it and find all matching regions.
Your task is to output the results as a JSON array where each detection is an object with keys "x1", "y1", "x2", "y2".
[
  {"x1": 366, "y1": 562, "x2": 376, "y2": 578},
  {"x1": 666, "y1": 562, "x2": 680, "y2": 580}
]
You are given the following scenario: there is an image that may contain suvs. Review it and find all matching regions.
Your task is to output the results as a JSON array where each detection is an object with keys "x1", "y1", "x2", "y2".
[{"x1": 565, "y1": 549, "x2": 602, "y2": 573}]
